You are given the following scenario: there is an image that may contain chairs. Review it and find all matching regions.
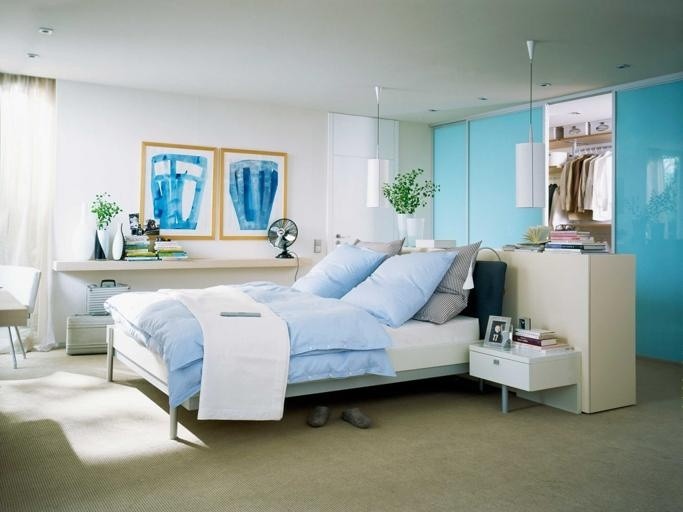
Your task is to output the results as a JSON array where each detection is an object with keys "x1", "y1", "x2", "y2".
[{"x1": 3, "y1": 264, "x2": 42, "y2": 369}]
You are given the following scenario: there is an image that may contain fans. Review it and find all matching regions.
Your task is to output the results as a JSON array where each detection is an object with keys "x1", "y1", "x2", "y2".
[{"x1": 265, "y1": 217, "x2": 299, "y2": 260}]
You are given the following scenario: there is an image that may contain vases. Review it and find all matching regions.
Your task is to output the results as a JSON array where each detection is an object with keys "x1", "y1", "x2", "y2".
[{"x1": 404, "y1": 218, "x2": 425, "y2": 244}]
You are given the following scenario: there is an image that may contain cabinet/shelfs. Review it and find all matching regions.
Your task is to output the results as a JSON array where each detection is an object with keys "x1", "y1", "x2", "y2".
[
  {"x1": 430, "y1": 70, "x2": 683, "y2": 367},
  {"x1": 492, "y1": 247, "x2": 638, "y2": 415}
]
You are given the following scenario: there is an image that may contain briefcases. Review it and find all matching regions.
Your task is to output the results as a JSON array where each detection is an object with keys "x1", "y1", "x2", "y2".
[
  {"x1": 66, "y1": 314, "x2": 115, "y2": 355},
  {"x1": 86, "y1": 279, "x2": 131, "y2": 316}
]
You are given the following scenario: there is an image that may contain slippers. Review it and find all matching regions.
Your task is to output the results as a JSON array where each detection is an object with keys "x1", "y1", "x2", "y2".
[
  {"x1": 342, "y1": 407, "x2": 371, "y2": 428},
  {"x1": 307, "y1": 406, "x2": 329, "y2": 426}
]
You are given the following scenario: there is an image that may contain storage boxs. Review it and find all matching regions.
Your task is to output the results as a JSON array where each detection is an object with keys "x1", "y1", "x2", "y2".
[{"x1": 549, "y1": 120, "x2": 612, "y2": 140}]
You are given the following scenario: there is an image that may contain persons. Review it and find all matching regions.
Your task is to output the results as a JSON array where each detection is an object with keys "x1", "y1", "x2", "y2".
[{"x1": 490, "y1": 324, "x2": 503, "y2": 342}]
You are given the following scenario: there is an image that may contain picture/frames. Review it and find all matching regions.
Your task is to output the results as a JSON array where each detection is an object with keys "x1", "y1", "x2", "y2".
[
  {"x1": 139, "y1": 138, "x2": 219, "y2": 242},
  {"x1": 218, "y1": 147, "x2": 288, "y2": 240}
]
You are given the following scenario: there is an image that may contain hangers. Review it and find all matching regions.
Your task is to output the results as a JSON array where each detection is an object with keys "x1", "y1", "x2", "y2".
[{"x1": 571, "y1": 143, "x2": 615, "y2": 158}]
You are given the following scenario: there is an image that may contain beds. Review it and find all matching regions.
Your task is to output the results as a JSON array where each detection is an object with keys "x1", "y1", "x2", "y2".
[{"x1": 104, "y1": 235, "x2": 508, "y2": 440}]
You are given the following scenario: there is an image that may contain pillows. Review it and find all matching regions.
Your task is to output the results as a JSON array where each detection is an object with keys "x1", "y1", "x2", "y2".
[
  {"x1": 340, "y1": 251, "x2": 459, "y2": 327},
  {"x1": 290, "y1": 243, "x2": 386, "y2": 300},
  {"x1": 413, "y1": 241, "x2": 483, "y2": 324},
  {"x1": 350, "y1": 237, "x2": 406, "y2": 256}
]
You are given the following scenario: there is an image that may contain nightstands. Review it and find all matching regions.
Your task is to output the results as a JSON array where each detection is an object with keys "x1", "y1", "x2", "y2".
[{"x1": 468, "y1": 342, "x2": 583, "y2": 417}]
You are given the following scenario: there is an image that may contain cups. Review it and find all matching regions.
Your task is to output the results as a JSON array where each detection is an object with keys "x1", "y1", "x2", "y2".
[{"x1": 501, "y1": 324, "x2": 513, "y2": 352}]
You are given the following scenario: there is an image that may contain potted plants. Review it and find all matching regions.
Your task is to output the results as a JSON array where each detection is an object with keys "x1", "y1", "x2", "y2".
[
  {"x1": 89, "y1": 192, "x2": 122, "y2": 260},
  {"x1": 381, "y1": 167, "x2": 441, "y2": 246}
]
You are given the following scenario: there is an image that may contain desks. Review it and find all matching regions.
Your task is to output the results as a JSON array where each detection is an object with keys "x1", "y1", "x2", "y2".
[{"x1": 0, "y1": 284, "x2": 29, "y2": 330}]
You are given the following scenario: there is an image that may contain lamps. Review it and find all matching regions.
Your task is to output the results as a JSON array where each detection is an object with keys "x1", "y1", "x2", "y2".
[
  {"x1": 515, "y1": 38, "x2": 546, "y2": 209},
  {"x1": 366, "y1": 84, "x2": 391, "y2": 208}
]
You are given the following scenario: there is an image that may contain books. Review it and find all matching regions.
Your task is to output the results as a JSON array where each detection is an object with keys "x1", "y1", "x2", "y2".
[
  {"x1": 124, "y1": 234, "x2": 188, "y2": 261},
  {"x1": 545, "y1": 230, "x2": 607, "y2": 254},
  {"x1": 512, "y1": 327, "x2": 569, "y2": 353}
]
